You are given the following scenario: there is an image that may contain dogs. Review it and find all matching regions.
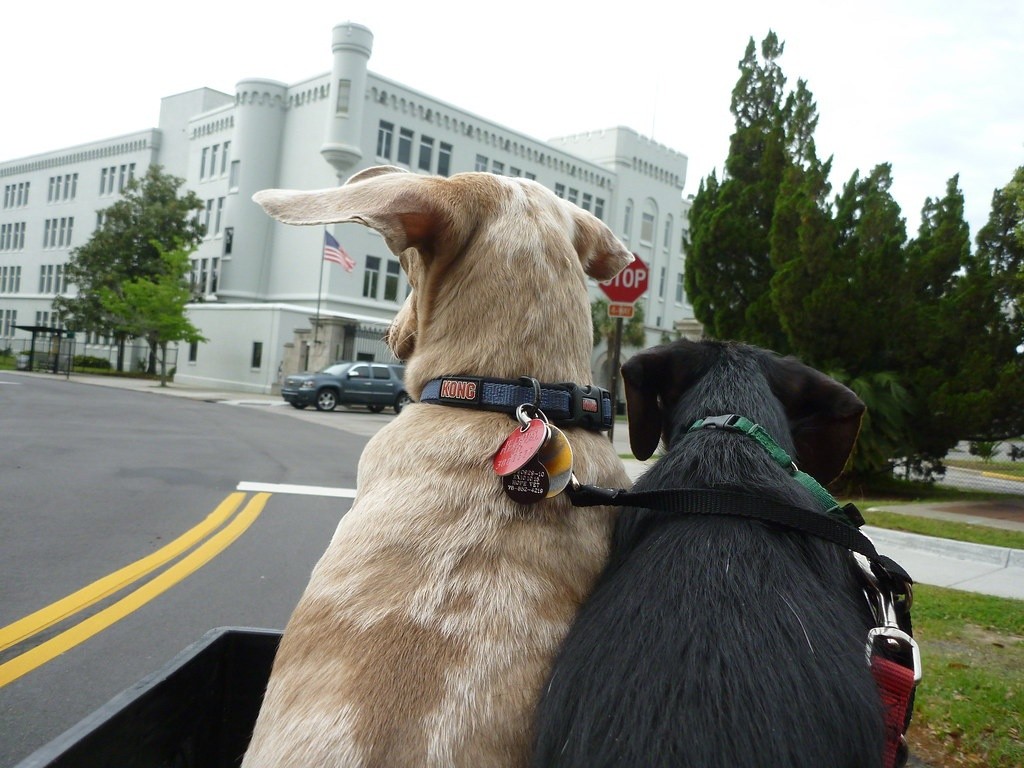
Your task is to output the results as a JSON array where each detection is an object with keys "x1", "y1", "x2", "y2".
[
  {"x1": 527, "y1": 338, "x2": 890, "y2": 767},
  {"x1": 239, "y1": 165, "x2": 636, "y2": 768}
]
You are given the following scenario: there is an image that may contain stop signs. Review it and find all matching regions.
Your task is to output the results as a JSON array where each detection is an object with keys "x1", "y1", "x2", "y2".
[{"x1": 597, "y1": 253, "x2": 649, "y2": 303}]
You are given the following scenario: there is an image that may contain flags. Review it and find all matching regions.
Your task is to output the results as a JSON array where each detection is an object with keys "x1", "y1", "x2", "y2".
[{"x1": 323, "y1": 230, "x2": 356, "y2": 273}]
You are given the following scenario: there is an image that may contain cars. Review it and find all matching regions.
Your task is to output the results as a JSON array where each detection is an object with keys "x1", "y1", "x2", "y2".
[{"x1": 282, "y1": 362, "x2": 414, "y2": 414}]
